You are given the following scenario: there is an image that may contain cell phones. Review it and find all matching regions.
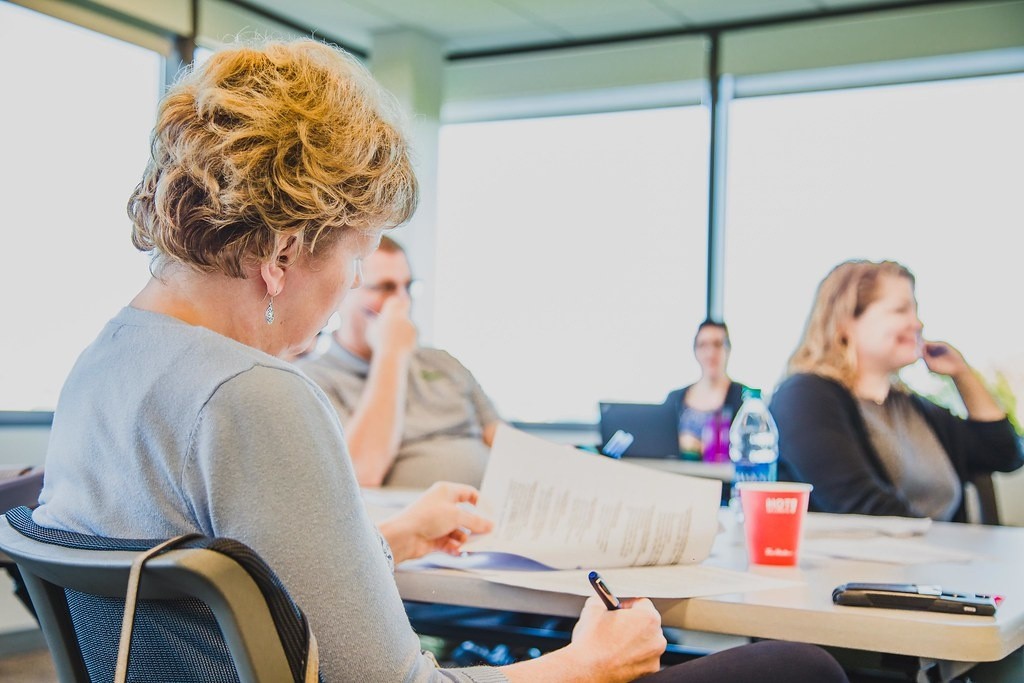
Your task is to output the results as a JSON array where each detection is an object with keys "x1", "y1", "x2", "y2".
[{"x1": 833, "y1": 583, "x2": 996, "y2": 617}]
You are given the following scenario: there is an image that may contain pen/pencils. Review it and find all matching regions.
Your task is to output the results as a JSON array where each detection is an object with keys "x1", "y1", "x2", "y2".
[{"x1": 587, "y1": 571, "x2": 619, "y2": 610}]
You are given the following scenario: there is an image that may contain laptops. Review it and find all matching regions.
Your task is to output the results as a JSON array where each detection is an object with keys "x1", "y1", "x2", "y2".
[{"x1": 599, "y1": 403, "x2": 681, "y2": 459}]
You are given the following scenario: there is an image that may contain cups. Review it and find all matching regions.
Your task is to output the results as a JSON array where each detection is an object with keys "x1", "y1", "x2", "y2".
[{"x1": 736, "y1": 481, "x2": 814, "y2": 566}]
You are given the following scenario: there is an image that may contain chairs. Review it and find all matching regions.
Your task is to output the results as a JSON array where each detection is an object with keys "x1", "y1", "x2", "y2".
[{"x1": 0, "y1": 506, "x2": 323, "y2": 683}]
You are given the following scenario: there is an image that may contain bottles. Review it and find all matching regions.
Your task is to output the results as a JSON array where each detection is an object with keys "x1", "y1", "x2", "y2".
[{"x1": 729, "y1": 387, "x2": 779, "y2": 546}]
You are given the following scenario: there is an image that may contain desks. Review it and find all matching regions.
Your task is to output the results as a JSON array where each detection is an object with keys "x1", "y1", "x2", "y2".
[{"x1": 393, "y1": 506, "x2": 1024, "y2": 683}]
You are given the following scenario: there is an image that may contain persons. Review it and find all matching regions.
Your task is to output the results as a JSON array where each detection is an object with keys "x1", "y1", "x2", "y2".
[
  {"x1": 30, "y1": 36, "x2": 852, "y2": 683},
  {"x1": 283, "y1": 234, "x2": 505, "y2": 493},
  {"x1": 769, "y1": 259, "x2": 1024, "y2": 522},
  {"x1": 662, "y1": 318, "x2": 750, "y2": 459}
]
